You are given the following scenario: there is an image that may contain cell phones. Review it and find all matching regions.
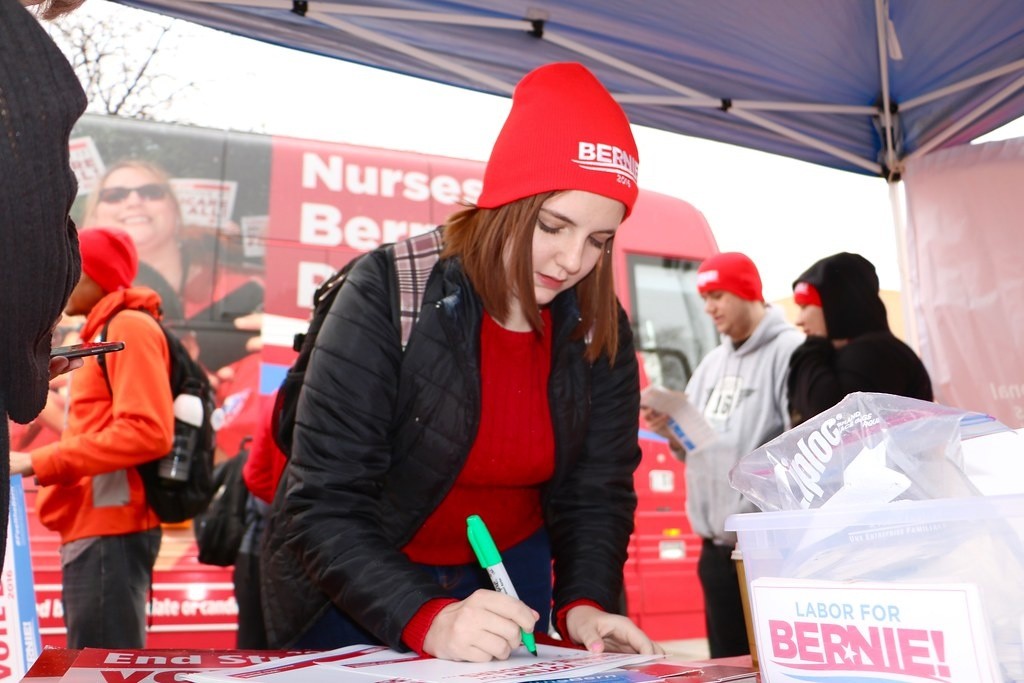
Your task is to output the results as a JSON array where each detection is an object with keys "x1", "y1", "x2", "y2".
[{"x1": 50, "y1": 342, "x2": 124, "y2": 360}]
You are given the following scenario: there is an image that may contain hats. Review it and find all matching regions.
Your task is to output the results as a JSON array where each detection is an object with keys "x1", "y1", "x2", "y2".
[
  {"x1": 474, "y1": 61, "x2": 640, "y2": 224},
  {"x1": 793, "y1": 281, "x2": 821, "y2": 310},
  {"x1": 696, "y1": 252, "x2": 765, "y2": 304},
  {"x1": 76, "y1": 224, "x2": 139, "y2": 293}
]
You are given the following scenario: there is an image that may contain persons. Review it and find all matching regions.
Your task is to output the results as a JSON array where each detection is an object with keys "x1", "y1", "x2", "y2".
[
  {"x1": 233, "y1": 62, "x2": 663, "y2": 661},
  {"x1": 42, "y1": 158, "x2": 261, "y2": 435},
  {"x1": 10, "y1": 226, "x2": 174, "y2": 651},
  {"x1": 644, "y1": 253, "x2": 807, "y2": 659},
  {"x1": 786, "y1": 252, "x2": 934, "y2": 429},
  {"x1": 0, "y1": 0, "x2": 89, "y2": 578}
]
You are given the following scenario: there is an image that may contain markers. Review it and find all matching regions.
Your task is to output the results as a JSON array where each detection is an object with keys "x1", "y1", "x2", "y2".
[{"x1": 466, "y1": 514, "x2": 539, "y2": 658}]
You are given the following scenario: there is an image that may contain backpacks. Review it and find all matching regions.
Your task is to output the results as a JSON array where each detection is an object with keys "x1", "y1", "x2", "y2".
[
  {"x1": 193, "y1": 447, "x2": 253, "y2": 565},
  {"x1": 273, "y1": 230, "x2": 445, "y2": 459},
  {"x1": 96, "y1": 305, "x2": 214, "y2": 524}
]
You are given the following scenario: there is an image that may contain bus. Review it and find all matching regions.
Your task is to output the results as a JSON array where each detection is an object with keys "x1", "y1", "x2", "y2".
[{"x1": 0, "y1": 115, "x2": 722, "y2": 659}]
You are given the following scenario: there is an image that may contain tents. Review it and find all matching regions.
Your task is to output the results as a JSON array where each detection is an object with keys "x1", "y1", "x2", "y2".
[{"x1": 106, "y1": 0, "x2": 1024, "y2": 320}]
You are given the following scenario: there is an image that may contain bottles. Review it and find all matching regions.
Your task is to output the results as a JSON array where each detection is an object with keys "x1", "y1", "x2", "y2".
[{"x1": 157, "y1": 393, "x2": 204, "y2": 482}]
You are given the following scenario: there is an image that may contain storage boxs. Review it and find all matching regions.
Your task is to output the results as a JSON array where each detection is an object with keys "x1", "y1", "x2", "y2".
[{"x1": 723, "y1": 493, "x2": 1024, "y2": 683}]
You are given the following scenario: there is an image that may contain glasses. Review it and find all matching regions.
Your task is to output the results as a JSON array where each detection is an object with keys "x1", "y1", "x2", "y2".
[{"x1": 99, "y1": 184, "x2": 167, "y2": 202}]
